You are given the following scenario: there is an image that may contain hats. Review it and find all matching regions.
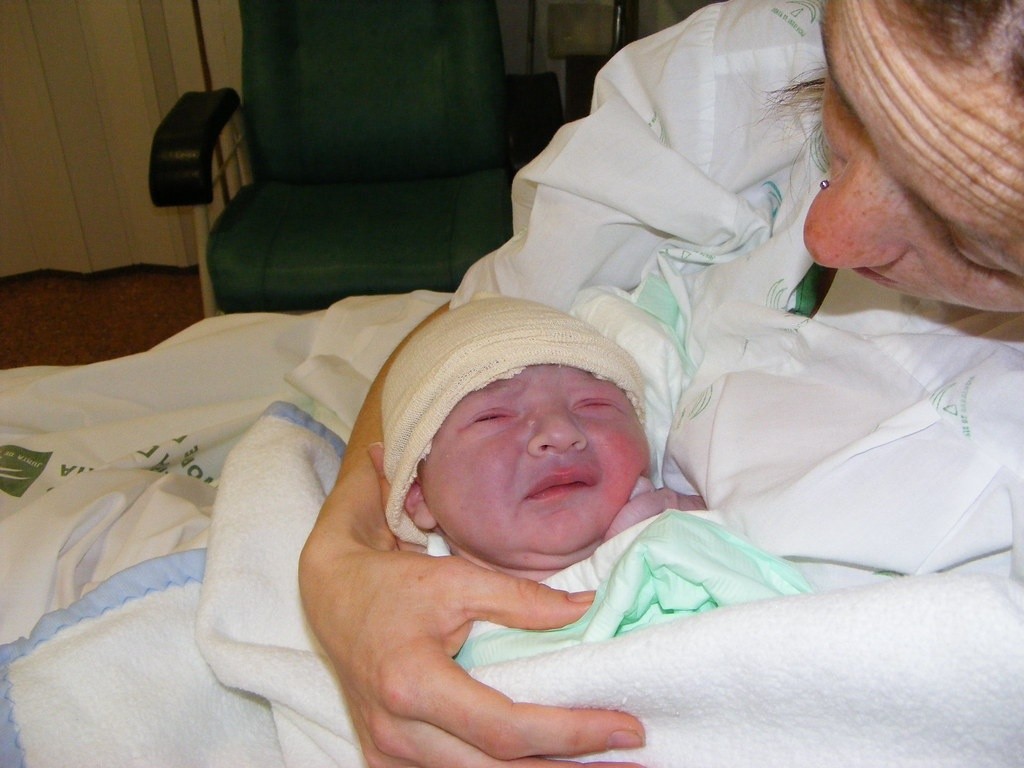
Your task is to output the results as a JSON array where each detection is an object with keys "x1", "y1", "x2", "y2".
[{"x1": 384, "y1": 296, "x2": 645, "y2": 546}]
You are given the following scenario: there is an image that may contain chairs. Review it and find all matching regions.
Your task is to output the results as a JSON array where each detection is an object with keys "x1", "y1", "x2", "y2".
[{"x1": 149, "y1": 1, "x2": 565, "y2": 320}]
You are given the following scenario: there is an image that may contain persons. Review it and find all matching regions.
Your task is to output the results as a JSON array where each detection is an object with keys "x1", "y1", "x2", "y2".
[
  {"x1": 291, "y1": 0, "x2": 1024, "y2": 768},
  {"x1": 383, "y1": 293, "x2": 818, "y2": 767}
]
